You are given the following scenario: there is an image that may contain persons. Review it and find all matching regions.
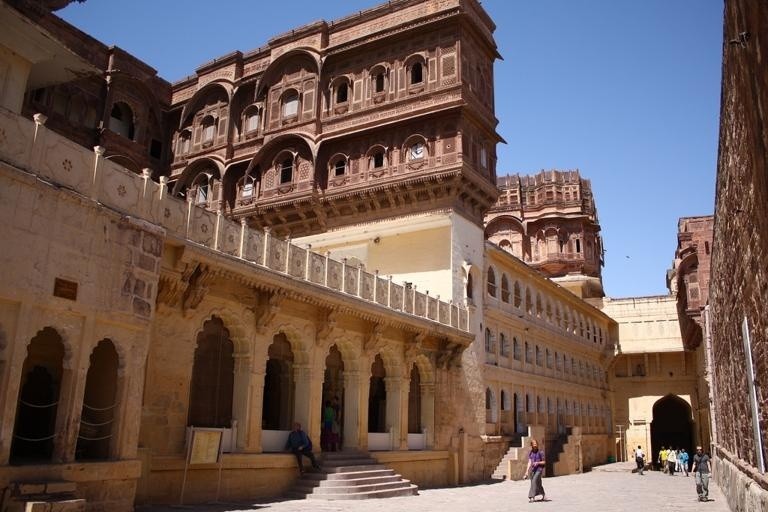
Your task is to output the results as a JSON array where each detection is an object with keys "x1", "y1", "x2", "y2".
[
  {"x1": 523, "y1": 439, "x2": 546, "y2": 502},
  {"x1": 691, "y1": 445, "x2": 712, "y2": 502},
  {"x1": 635, "y1": 445, "x2": 645, "y2": 475},
  {"x1": 285, "y1": 423, "x2": 320, "y2": 473},
  {"x1": 658, "y1": 446, "x2": 690, "y2": 477},
  {"x1": 325, "y1": 396, "x2": 342, "y2": 452}
]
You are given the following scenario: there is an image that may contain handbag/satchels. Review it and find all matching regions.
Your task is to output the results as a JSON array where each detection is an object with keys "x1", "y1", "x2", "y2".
[{"x1": 694, "y1": 465, "x2": 699, "y2": 473}]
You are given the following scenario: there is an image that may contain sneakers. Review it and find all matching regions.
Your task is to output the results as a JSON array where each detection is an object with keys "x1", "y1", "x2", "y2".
[{"x1": 698, "y1": 495, "x2": 708, "y2": 502}]
